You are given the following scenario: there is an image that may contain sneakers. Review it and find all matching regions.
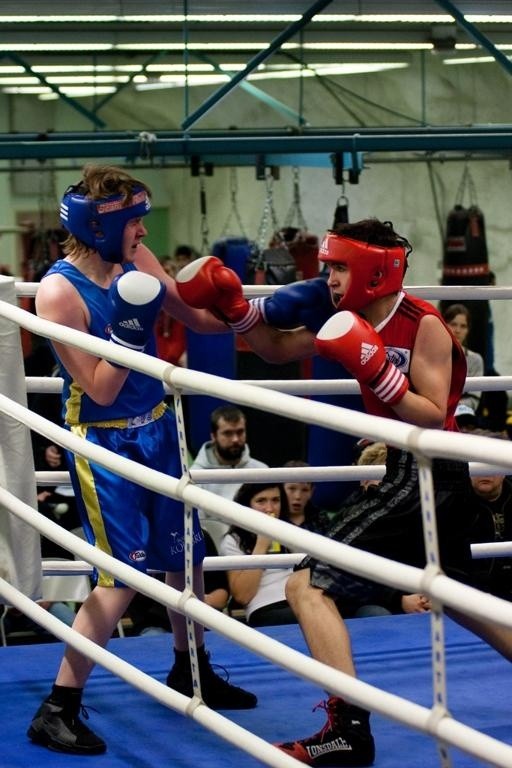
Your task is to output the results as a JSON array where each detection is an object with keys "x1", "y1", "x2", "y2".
[
  {"x1": 270, "y1": 700, "x2": 375, "y2": 767},
  {"x1": 26, "y1": 700, "x2": 105, "y2": 756},
  {"x1": 166, "y1": 651, "x2": 258, "y2": 711}
]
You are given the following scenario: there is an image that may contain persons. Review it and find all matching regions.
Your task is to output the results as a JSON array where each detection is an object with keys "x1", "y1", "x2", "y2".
[
  {"x1": 175, "y1": 217, "x2": 510, "y2": 767},
  {"x1": 25, "y1": 159, "x2": 339, "y2": 757}
]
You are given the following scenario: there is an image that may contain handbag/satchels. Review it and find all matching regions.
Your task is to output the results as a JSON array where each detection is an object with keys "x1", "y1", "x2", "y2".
[{"x1": 107, "y1": 270, "x2": 167, "y2": 350}]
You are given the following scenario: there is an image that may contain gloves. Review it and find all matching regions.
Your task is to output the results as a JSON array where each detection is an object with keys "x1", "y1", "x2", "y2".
[
  {"x1": 315, "y1": 309, "x2": 410, "y2": 405},
  {"x1": 175, "y1": 255, "x2": 335, "y2": 335}
]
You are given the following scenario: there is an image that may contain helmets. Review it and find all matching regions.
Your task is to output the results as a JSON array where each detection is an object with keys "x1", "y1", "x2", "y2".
[
  {"x1": 59, "y1": 185, "x2": 153, "y2": 264},
  {"x1": 317, "y1": 232, "x2": 406, "y2": 311}
]
